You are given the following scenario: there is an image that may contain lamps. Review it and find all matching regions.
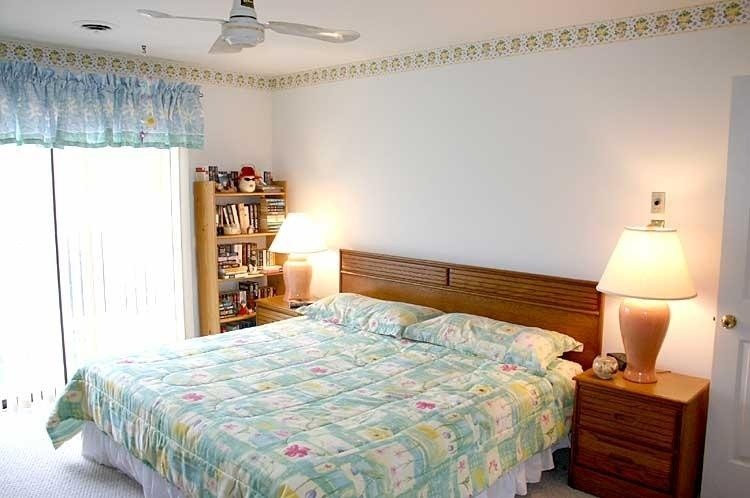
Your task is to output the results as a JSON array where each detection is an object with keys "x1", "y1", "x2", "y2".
[
  {"x1": 266, "y1": 211, "x2": 329, "y2": 302},
  {"x1": 590, "y1": 224, "x2": 698, "y2": 387}
]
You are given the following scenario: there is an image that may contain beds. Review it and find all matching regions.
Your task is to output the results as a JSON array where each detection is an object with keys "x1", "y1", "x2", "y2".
[{"x1": 38, "y1": 245, "x2": 607, "y2": 498}]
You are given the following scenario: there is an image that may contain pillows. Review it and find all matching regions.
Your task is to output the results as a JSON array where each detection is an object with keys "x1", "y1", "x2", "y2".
[
  {"x1": 400, "y1": 311, "x2": 585, "y2": 378},
  {"x1": 294, "y1": 293, "x2": 444, "y2": 342}
]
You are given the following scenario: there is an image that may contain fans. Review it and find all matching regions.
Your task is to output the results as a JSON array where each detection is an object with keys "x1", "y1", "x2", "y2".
[{"x1": 136, "y1": 0, "x2": 361, "y2": 55}]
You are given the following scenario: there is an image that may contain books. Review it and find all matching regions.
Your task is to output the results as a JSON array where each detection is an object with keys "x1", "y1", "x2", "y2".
[{"x1": 196, "y1": 165, "x2": 285, "y2": 333}]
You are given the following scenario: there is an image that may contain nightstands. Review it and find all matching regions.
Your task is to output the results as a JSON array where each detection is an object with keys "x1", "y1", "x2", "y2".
[
  {"x1": 566, "y1": 365, "x2": 711, "y2": 498},
  {"x1": 253, "y1": 292, "x2": 324, "y2": 329}
]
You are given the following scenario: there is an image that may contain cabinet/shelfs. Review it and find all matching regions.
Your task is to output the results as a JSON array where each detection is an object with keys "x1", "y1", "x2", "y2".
[{"x1": 191, "y1": 177, "x2": 288, "y2": 336}]
